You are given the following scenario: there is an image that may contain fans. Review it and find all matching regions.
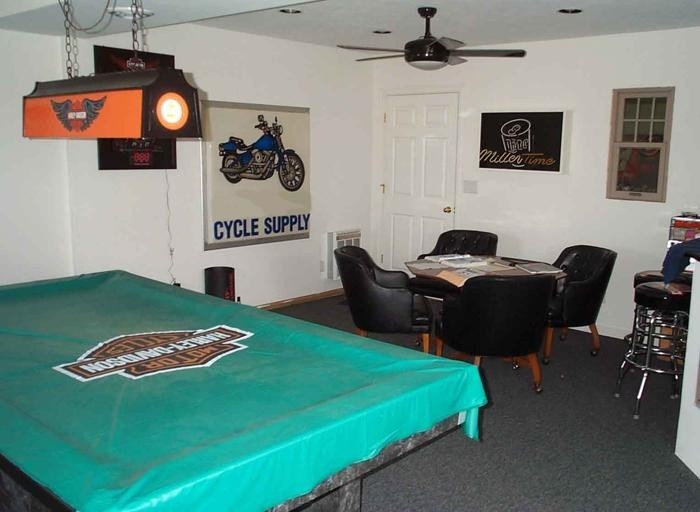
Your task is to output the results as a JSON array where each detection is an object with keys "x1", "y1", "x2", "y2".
[{"x1": 337, "y1": 5, "x2": 525, "y2": 69}]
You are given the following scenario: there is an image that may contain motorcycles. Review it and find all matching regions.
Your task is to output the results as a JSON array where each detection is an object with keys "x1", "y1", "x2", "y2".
[{"x1": 214, "y1": 111, "x2": 307, "y2": 193}]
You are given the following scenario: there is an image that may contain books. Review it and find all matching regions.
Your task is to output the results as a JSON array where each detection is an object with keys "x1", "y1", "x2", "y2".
[{"x1": 408, "y1": 253, "x2": 564, "y2": 288}]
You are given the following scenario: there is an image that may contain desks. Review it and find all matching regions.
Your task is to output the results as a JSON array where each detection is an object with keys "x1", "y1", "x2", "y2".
[
  {"x1": 0, "y1": 271, "x2": 489, "y2": 512},
  {"x1": 408, "y1": 255, "x2": 567, "y2": 356}
]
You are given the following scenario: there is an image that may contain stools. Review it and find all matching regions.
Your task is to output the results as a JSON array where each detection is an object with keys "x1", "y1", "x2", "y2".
[
  {"x1": 616, "y1": 281, "x2": 691, "y2": 422},
  {"x1": 622, "y1": 270, "x2": 694, "y2": 398}
]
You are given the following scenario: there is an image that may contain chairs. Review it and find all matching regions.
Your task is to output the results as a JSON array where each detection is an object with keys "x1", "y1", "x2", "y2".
[
  {"x1": 542, "y1": 243, "x2": 617, "y2": 365},
  {"x1": 435, "y1": 274, "x2": 556, "y2": 393},
  {"x1": 416, "y1": 230, "x2": 498, "y2": 260},
  {"x1": 334, "y1": 244, "x2": 432, "y2": 355}
]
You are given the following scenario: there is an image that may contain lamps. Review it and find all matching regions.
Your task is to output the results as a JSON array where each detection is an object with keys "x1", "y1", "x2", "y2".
[{"x1": 24, "y1": 1, "x2": 202, "y2": 140}]
